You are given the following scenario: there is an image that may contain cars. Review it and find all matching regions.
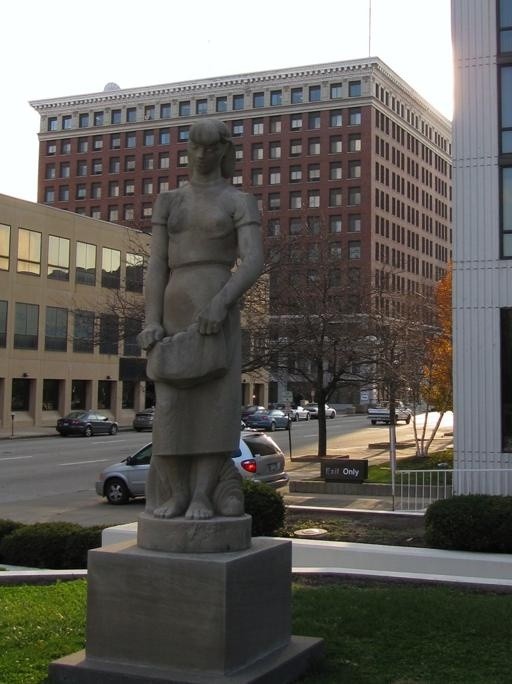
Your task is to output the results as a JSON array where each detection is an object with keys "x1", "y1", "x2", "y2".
[
  {"x1": 241, "y1": 403, "x2": 337, "y2": 431},
  {"x1": 134, "y1": 406, "x2": 154, "y2": 433},
  {"x1": 96, "y1": 431, "x2": 288, "y2": 505},
  {"x1": 58, "y1": 410, "x2": 119, "y2": 437}
]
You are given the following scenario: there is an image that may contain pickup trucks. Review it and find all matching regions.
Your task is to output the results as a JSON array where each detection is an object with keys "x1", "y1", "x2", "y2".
[{"x1": 368, "y1": 399, "x2": 412, "y2": 425}]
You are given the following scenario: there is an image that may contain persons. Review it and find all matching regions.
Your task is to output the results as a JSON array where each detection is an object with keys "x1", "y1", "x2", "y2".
[{"x1": 134, "y1": 115, "x2": 268, "y2": 527}]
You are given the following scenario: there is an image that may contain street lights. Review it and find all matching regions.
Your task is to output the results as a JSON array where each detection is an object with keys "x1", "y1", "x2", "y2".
[{"x1": 388, "y1": 360, "x2": 400, "y2": 496}]
